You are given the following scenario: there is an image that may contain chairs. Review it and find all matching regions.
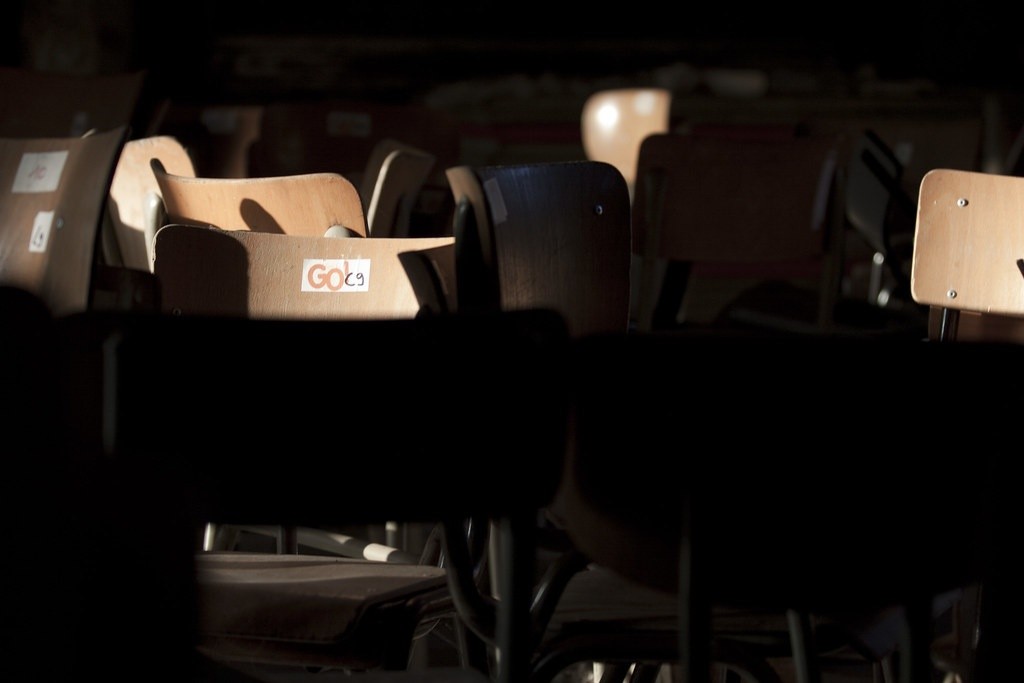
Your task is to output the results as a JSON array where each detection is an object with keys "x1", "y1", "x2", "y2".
[
  {"x1": 446, "y1": 159, "x2": 899, "y2": 683},
  {"x1": 577, "y1": 78, "x2": 673, "y2": 209},
  {"x1": 157, "y1": 172, "x2": 374, "y2": 237},
  {"x1": 0, "y1": 59, "x2": 149, "y2": 317},
  {"x1": 359, "y1": 133, "x2": 431, "y2": 237},
  {"x1": 908, "y1": 168, "x2": 1024, "y2": 340},
  {"x1": 142, "y1": 225, "x2": 453, "y2": 683},
  {"x1": 634, "y1": 131, "x2": 845, "y2": 337}
]
